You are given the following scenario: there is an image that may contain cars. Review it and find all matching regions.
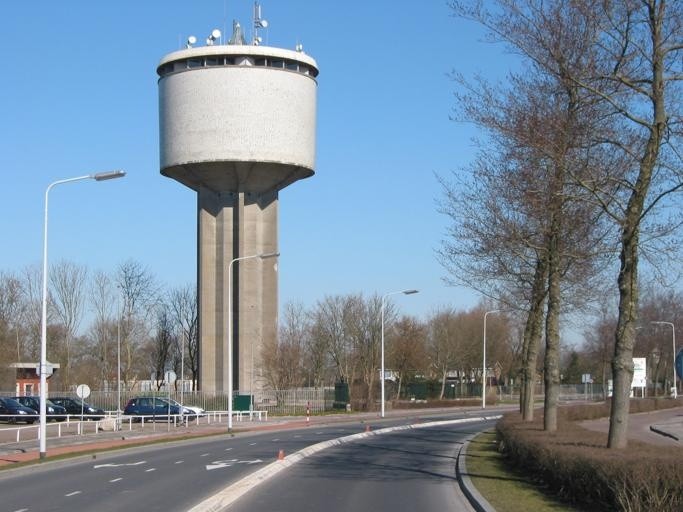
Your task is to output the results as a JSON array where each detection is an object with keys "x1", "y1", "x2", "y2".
[{"x1": 0, "y1": 394, "x2": 205, "y2": 425}]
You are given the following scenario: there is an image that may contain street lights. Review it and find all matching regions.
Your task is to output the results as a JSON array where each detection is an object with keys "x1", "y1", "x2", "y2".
[
  {"x1": 37, "y1": 167, "x2": 127, "y2": 460},
  {"x1": 650, "y1": 320, "x2": 677, "y2": 400},
  {"x1": 378, "y1": 288, "x2": 418, "y2": 419},
  {"x1": 224, "y1": 251, "x2": 280, "y2": 431},
  {"x1": 481, "y1": 308, "x2": 499, "y2": 410}
]
